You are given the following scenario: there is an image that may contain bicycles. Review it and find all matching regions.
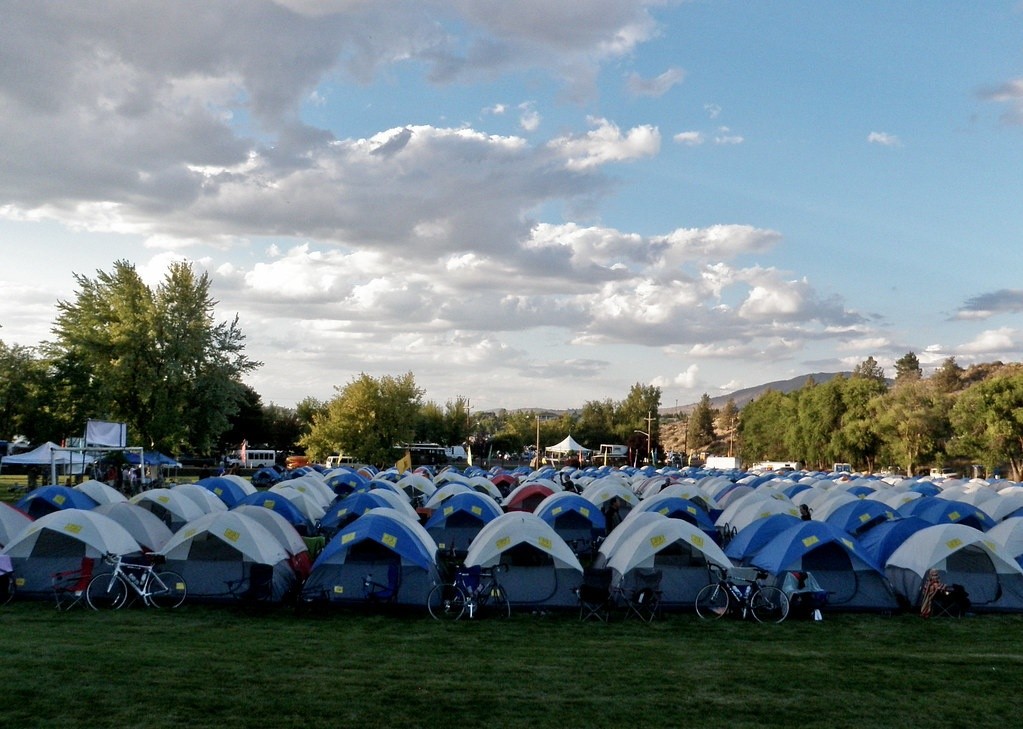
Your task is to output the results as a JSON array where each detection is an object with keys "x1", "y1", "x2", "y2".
[
  {"x1": 0, "y1": 568, "x2": 17, "y2": 609},
  {"x1": 86, "y1": 550, "x2": 187, "y2": 612},
  {"x1": 427, "y1": 562, "x2": 511, "y2": 625},
  {"x1": 695, "y1": 560, "x2": 790, "y2": 626}
]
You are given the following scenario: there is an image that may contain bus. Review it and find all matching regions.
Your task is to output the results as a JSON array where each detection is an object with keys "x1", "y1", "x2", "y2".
[
  {"x1": 326, "y1": 456, "x2": 361, "y2": 469},
  {"x1": 227, "y1": 449, "x2": 275, "y2": 469}
]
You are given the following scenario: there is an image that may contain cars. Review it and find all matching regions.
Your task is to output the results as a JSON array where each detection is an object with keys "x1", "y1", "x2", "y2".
[{"x1": 503, "y1": 444, "x2": 537, "y2": 462}]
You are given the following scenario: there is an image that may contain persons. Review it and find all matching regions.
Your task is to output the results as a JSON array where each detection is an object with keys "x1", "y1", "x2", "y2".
[
  {"x1": 28, "y1": 460, "x2": 147, "y2": 497},
  {"x1": 560, "y1": 475, "x2": 574, "y2": 491},
  {"x1": 606, "y1": 497, "x2": 623, "y2": 537},
  {"x1": 799, "y1": 504, "x2": 812, "y2": 521}
]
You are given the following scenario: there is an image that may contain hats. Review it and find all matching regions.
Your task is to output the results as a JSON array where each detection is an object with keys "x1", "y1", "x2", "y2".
[{"x1": 665, "y1": 477, "x2": 670, "y2": 483}]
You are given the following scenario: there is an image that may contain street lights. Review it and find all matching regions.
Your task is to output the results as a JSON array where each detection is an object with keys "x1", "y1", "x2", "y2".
[
  {"x1": 634, "y1": 410, "x2": 656, "y2": 459},
  {"x1": 462, "y1": 397, "x2": 475, "y2": 454}
]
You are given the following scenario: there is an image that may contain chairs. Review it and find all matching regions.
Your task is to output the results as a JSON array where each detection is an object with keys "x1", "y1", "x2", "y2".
[
  {"x1": 573, "y1": 567, "x2": 665, "y2": 625},
  {"x1": 922, "y1": 570, "x2": 968, "y2": 620},
  {"x1": 782, "y1": 569, "x2": 838, "y2": 622},
  {"x1": 361, "y1": 562, "x2": 403, "y2": 608},
  {"x1": 227, "y1": 536, "x2": 326, "y2": 611},
  {"x1": 44, "y1": 557, "x2": 97, "y2": 611},
  {"x1": 450, "y1": 565, "x2": 482, "y2": 613}
]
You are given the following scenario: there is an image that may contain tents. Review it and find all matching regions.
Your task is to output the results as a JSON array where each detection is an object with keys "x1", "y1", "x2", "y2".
[
  {"x1": 545, "y1": 433, "x2": 592, "y2": 452},
  {"x1": 0, "y1": 440, "x2": 182, "y2": 486},
  {"x1": 0, "y1": 465, "x2": 1023, "y2": 609}
]
